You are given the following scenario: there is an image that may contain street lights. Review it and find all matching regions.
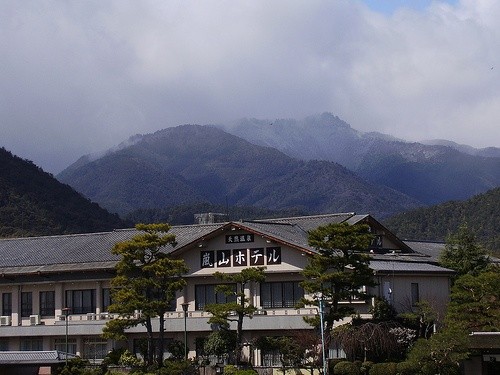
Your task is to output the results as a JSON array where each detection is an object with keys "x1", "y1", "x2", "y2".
[
  {"x1": 179, "y1": 303, "x2": 190, "y2": 361},
  {"x1": 62, "y1": 307, "x2": 72, "y2": 366},
  {"x1": 312, "y1": 292, "x2": 328, "y2": 375}
]
